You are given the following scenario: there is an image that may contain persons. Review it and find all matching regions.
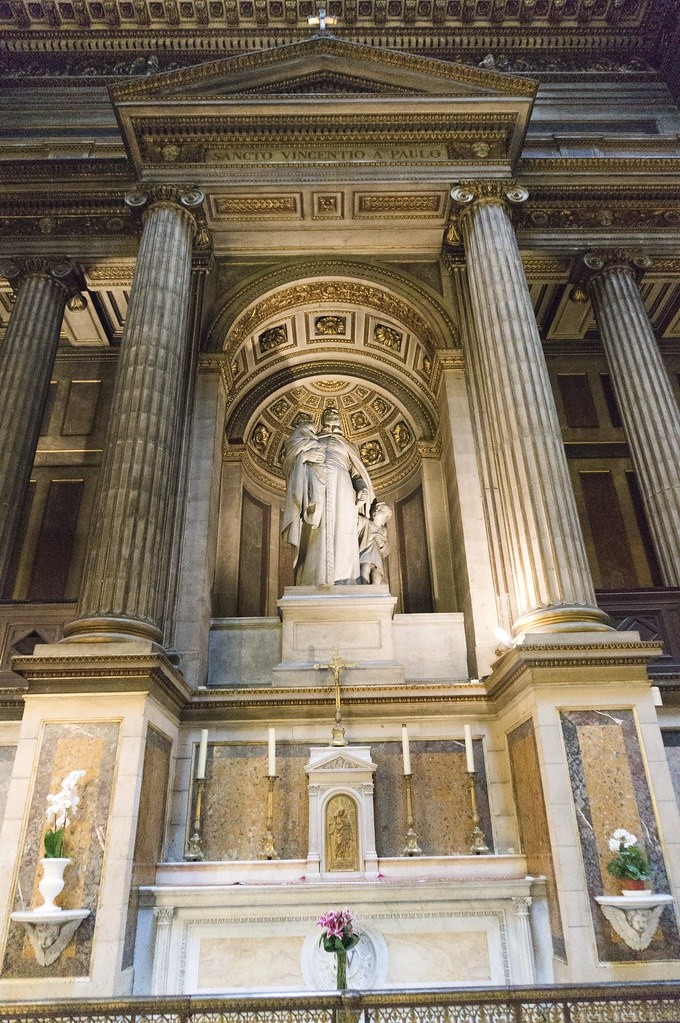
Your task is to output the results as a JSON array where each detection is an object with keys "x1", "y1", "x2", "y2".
[
  {"x1": 280, "y1": 408, "x2": 393, "y2": 586},
  {"x1": 329, "y1": 665, "x2": 345, "y2": 678},
  {"x1": 329, "y1": 807, "x2": 350, "y2": 858}
]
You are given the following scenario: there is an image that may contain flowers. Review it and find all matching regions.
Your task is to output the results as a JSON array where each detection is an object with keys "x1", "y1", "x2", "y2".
[
  {"x1": 315, "y1": 908, "x2": 363, "y2": 990},
  {"x1": 42, "y1": 768, "x2": 87, "y2": 859},
  {"x1": 604, "y1": 827, "x2": 657, "y2": 881}
]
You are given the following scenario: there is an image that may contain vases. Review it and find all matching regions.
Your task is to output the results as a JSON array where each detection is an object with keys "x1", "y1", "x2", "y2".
[
  {"x1": 620, "y1": 876, "x2": 654, "y2": 896},
  {"x1": 32, "y1": 857, "x2": 72, "y2": 913}
]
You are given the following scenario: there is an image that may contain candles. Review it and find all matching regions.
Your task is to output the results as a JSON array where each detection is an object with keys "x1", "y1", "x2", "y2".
[
  {"x1": 463, "y1": 723, "x2": 474, "y2": 772},
  {"x1": 268, "y1": 725, "x2": 276, "y2": 776},
  {"x1": 197, "y1": 728, "x2": 208, "y2": 778},
  {"x1": 401, "y1": 726, "x2": 412, "y2": 775}
]
